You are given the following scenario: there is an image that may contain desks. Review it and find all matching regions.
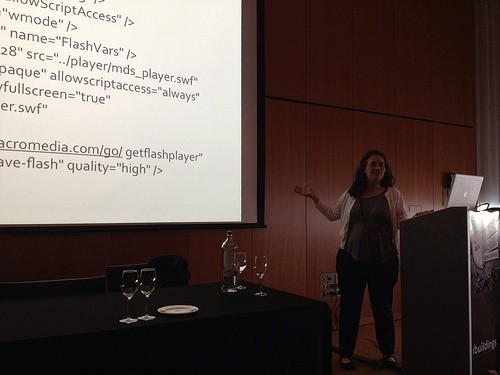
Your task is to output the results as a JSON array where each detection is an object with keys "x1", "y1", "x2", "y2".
[{"x1": 0, "y1": 279, "x2": 336, "y2": 375}]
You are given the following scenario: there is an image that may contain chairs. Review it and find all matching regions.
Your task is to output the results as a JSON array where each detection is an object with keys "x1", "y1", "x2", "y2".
[
  {"x1": 105, "y1": 262, "x2": 148, "y2": 289},
  {"x1": 0, "y1": 275, "x2": 108, "y2": 300},
  {"x1": 146, "y1": 254, "x2": 192, "y2": 287}
]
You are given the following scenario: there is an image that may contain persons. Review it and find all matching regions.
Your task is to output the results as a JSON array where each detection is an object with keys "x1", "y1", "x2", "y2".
[{"x1": 292, "y1": 150, "x2": 434, "y2": 369}]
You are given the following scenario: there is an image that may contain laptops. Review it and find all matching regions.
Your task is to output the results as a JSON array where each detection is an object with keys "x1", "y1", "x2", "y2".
[{"x1": 447, "y1": 174, "x2": 484, "y2": 211}]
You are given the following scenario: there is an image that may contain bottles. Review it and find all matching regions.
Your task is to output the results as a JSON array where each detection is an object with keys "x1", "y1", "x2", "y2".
[{"x1": 220, "y1": 230, "x2": 238, "y2": 293}]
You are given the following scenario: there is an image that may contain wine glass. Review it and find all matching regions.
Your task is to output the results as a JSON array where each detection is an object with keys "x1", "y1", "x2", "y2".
[
  {"x1": 118, "y1": 269, "x2": 138, "y2": 323},
  {"x1": 234, "y1": 252, "x2": 247, "y2": 290},
  {"x1": 253, "y1": 255, "x2": 268, "y2": 296},
  {"x1": 138, "y1": 268, "x2": 156, "y2": 321}
]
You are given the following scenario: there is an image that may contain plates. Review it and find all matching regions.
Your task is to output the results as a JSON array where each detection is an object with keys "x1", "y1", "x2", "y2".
[{"x1": 158, "y1": 305, "x2": 198, "y2": 315}]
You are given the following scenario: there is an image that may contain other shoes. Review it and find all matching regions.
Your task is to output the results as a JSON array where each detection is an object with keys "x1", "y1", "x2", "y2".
[
  {"x1": 339, "y1": 357, "x2": 353, "y2": 370},
  {"x1": 383, "y1": 354, "x2": 398, "y2": 368}
]
